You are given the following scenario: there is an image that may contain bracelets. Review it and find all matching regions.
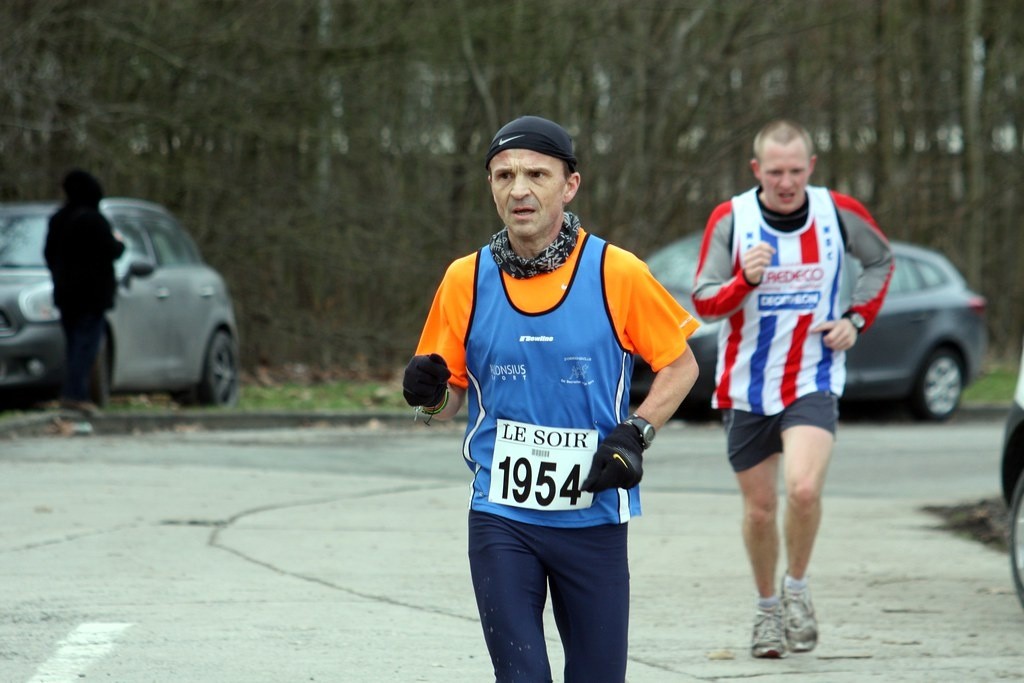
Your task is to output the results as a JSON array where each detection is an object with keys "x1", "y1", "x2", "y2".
[{"x1": 414, "y1": 388, "x2": 449, "y2": 426}]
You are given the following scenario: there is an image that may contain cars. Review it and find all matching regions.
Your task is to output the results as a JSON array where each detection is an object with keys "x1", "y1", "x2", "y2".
[
  {"x1": 630, "y1": 233, "x2": 991, "y2": 426},
  {"x1": 1002, "y1": 359, "x2": 1024, "y2": 609},
  {"x1": 1, "y1": 200, "x2": 236, "y2": 415}
]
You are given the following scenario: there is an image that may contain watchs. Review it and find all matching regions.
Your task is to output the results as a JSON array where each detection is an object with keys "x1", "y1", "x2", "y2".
[
  {"x1": 842, "y1": 311, "x2": 865, "y2": 334},
  {"x1": 625, "y1": 413, "x2": 655, "y2": 446}
]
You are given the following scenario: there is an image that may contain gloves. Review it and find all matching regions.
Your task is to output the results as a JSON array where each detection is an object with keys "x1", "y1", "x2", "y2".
[
  {"x1": 403, "y1": 353, "x2": 451, "y2": 407},
  {"x1": 580, "y1": 423, "x2": 645, "y2": 493}
]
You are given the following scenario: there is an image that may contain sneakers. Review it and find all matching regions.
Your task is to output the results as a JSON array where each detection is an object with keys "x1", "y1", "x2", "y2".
[
  {"x1": 751, "y1": 596, "x2": 789, "y2": 658},
  {"x1": 781, "y1": 570, "x2": 817, "y2": 651}
]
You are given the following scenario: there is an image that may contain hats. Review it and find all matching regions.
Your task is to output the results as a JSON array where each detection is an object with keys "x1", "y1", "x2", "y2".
[{"x1": 484, "y1": 115, "x2": 578, "y2": 172}]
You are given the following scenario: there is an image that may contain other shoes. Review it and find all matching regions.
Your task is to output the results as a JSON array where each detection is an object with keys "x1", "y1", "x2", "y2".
[
  {"x1": 57, "y1": 420, "x2": 93, "y2": 435},
  {"x1": 67, "y1": 398, "x2": 102, "y2": 417}
]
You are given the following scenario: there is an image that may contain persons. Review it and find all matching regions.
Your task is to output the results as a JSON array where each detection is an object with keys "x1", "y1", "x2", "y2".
[
  {"x1": 45, "y1": 170, "x2": 123, "y2": 414},
  {"x1": 691, "y1": 117, "x2": 896, "y2": 658},
  {"x1": 401, "y1": 116, "x2": 700, "y2": 683}
]
